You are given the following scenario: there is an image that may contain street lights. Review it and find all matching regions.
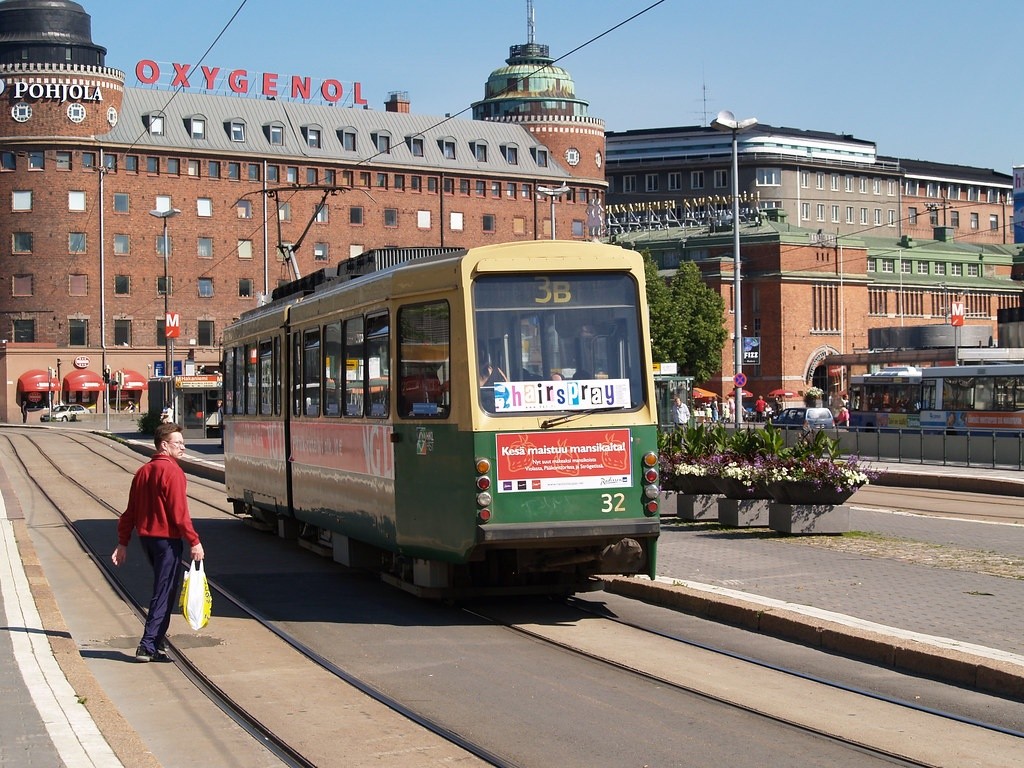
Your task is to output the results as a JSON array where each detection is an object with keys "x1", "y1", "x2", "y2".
[
  {"x1": 710, "y1": 116, "x2": 759, "y2": 431},
  {"x1": 538, "y1": 184, "x2": 571, "y2": 240},
  {"x1": 149, "y1": 209, "x2": 181, "y2": 377}
]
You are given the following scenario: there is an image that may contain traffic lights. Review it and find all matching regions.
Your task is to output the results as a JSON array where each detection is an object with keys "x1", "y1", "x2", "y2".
[{"x1": 110, "y1": 380, "x2": 119, "y2": 386}]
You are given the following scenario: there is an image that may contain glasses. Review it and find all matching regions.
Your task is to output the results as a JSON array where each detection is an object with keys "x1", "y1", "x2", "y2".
[{"x1": 167, "y1": 441, "x2": 186, "y2": 447}]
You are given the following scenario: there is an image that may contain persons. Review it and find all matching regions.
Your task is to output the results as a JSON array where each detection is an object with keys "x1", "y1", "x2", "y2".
[
  {"x1": 756, "y1": 396, "x2": 772, "y2": 424},
  {"x1": 837, "y1": 394, "x2": 849, "y2": 427},
  {"x1": 947, "y1": 413, "x2": 955, "y2": 426},
  {"x1": 775, "y1": 396, "x2": 783, "y2": 414},
  {"x1": 478, "y1": 340, "x2": 503, "y2": 391},
  {"x1": 217, "y1": 400, "x2": 224, "y2": 449},
  {"x1": 723, "y1": 395, "x2": 735, "y2": 423},
  {"x1": 112, "y1": 423, "x2": 204, "y2": 662},
  {"x1": 703, "y1": 396, "x2": 719, "y2": 423},
  {"x1": 672, "y1": 398, "x2": 690, "y2": 434},
  {"x1": 124, "y1": 401, "x2": 135, "y2": 413},
  {"x1": 166, "y1": 403, "x2": 173, "y2": 424}
]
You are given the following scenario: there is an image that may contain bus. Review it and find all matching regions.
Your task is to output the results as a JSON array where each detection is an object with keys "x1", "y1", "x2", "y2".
[
  {"x1": 843, "y1": 364, "x2": 1024, "y2": 438},
  {"x1": 195, "y1": 185, "x2": 661, "y2": 606}
]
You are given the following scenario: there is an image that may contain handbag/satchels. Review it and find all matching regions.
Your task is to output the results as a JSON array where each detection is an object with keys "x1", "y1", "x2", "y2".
[
  {"x1": 710, "y1": 403, "x2": 716, "y2": 410},
  {"x1": 178, "y1": 559, "x2": 213, "y2": 630}
]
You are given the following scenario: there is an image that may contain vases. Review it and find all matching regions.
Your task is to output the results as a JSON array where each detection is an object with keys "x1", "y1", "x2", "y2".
[
  {"x1": 674, "y1": 475, "x2": 720, "y2": 494},
  {"x1": 763, "y1": 480, "x2": 866, "y2": 505},
  {"x1": 709, "y1": 475, "x2": 772, "y2": 500},
  {"x1": 660, "y1": 476, "x2": 677, "y2": 491}
]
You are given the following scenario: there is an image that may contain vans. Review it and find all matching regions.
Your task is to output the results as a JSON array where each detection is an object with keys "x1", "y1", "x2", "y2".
[{"x1": 762, "y1": 407, "x2": 837, "y2": 430}]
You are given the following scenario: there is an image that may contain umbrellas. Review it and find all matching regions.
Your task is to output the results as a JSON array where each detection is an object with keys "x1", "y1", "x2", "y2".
[
  {"x1": 693, "y1": 388, "x2": 717, "y2": 399},
  {"x1": 767, "y1": 389, "x2": 800, "y2": 397},
  {"x1": 729, "y1": 390, "x2": 753, "y2": 397}
]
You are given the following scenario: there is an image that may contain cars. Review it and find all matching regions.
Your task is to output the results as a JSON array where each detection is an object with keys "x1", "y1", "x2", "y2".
[{"x1": 40, "y1": 404, "x2": 91, "y2": 422}]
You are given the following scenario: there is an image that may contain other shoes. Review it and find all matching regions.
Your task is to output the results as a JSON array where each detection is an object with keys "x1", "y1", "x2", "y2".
[
  {"x1": 135, "y1": 646, "x2": 167, "y2": 661},
  {"x1": 158, "y1": 642, "x2": 166, "y2": 651}
]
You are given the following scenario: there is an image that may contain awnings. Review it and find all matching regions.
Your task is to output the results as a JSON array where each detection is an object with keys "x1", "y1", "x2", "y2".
[
  {"x1": 64, "y1": 369, "x2": 106, "y2": 392},
  {"x1": 18, "y1": 369, "x2": 61, "y2": 392},
  {"x1": 110, "y1": 370, "x2": 149, "y2": 390}
]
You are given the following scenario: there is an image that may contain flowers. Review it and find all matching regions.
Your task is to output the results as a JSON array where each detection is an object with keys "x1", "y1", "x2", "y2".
[{"x1": 658, "y1": 425, "x2": 888, "y2": 493}]
